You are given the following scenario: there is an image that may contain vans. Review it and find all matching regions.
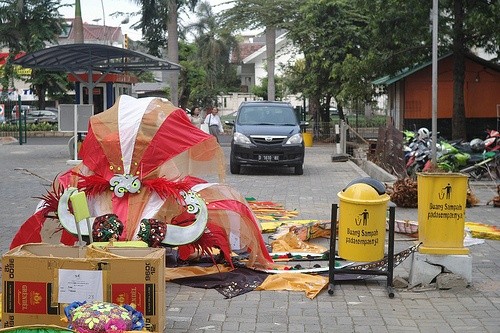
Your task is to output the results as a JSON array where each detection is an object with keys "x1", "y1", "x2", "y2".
[
  {"x1": 11, "y1": 104, "x2": 37, "y2": 119},
  {"x1": 225, "y1": 99, "x2": 309, "y2": 176}
]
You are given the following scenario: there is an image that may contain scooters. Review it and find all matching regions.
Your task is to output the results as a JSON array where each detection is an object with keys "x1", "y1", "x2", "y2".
[{"x1": 399, "y1": 129, "x2": 500, "y2": 181}]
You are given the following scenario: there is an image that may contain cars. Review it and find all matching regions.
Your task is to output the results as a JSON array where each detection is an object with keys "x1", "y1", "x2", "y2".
[{"x1": 5, "y1": 110, "x2": 58, "y2": 126}]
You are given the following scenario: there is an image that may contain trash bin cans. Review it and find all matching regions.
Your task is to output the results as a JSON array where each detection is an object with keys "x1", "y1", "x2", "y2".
[
  {"x1": 416, "y1": 172, "x2": 470, "y2": 256},
  {"x1": 303, "y1": 133, "x2": 313, "y2": 147},
  {"x1": 338, "y1": 179, "x2": 391, "y2": 262}
]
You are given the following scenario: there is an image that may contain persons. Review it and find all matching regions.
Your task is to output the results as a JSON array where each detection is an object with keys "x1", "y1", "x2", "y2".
[
  {"x1": 190, "y1": 107, "x2": 204, "y2": 129},
  {"x1": 204, "y1": 107, "x2": 224, "y2": 142}
]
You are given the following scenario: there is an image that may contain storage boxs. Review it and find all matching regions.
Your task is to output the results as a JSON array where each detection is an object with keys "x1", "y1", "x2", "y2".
[{"x1": 0, "y1": 241, "x2": 167, "y2": 333}]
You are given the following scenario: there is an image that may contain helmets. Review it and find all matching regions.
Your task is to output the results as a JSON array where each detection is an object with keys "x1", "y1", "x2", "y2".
[
  {"x1": 470, "y1": 138, "x2": 485, "y2": 149},
  {"x1": 418, "y1": 128, "x2": 430, "y2": 140}
]
